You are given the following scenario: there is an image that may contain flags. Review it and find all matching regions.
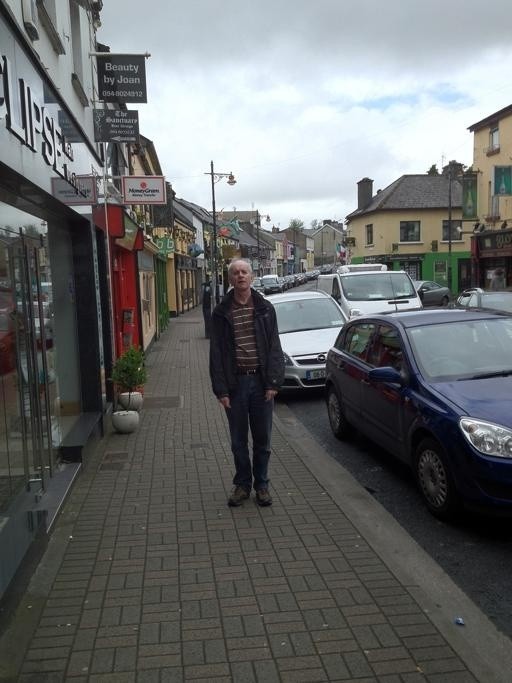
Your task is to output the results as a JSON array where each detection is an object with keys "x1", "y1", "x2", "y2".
[
  {"x1": 335, "y1": 241, "x2": 347, "y2": 258},
  {"x1": 225, "y1": 214, "x2": 241, "y2": 237}
]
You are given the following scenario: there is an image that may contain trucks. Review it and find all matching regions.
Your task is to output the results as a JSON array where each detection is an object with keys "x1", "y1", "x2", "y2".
[
  {"x1": 314, "y1": 269, "x2": 424, "y2": 321},
  {"x1": 335, "y1": 263, "x2": 388, "y2": 274}
]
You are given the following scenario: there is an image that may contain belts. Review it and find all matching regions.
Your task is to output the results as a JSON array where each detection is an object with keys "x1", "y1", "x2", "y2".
[{"x1": 243, "y1": 369, "x2": 258, "y2": 376}]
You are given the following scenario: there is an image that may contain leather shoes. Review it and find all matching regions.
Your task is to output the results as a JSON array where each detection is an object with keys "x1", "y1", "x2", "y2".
[
  {"x1": 228, "y1": 486, "x2": 249, "y2": 506},
  {"x1": 256, "y1": 487, "x2": 272, "y2": 506}
]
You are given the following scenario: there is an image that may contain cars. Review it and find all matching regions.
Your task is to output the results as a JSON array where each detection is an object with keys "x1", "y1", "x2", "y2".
[
  {"x1": 0, "y1": 277, "x2": 52, "y2": 377},
  {"x1": 411, "y1": 279, "x2": 452, "y2": 307},
  {"x1": 223, "y1": 263, "x2": 333, "y2": 303},
  {"x1": 325, "y1": 306, "x2": 512, "y2": 524},
  {"x1": 254, "y1": 287, "x2": 352, "y2": 392}
]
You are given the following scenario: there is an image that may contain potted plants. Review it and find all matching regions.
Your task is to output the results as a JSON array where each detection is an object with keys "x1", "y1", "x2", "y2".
[{"x1": 106, "y1": 344, "x2": 152, "y2": 434}]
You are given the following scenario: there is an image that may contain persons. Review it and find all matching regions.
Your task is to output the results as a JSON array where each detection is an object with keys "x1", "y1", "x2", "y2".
[
  {"x1": 209, "y1": 257, "x2": 285, "y2": 506},
  {"x1": 199, "y1": 273, "x2": 215, "y2": 339},
  {"x1": 489, "y1": 266, "x2": 506, "y2": 291}
]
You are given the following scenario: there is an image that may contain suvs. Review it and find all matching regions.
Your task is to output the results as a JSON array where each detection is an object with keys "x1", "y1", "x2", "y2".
[{"x1": 455, "y1": 286, "x2": 512, "y2": 313}]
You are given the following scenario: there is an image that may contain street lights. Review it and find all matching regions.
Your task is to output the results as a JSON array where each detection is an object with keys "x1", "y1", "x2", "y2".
[
  {"x1": 249, "y1": 208, "x2": 272, "y2": 276},
  {"x1": 293, "y1": 227, "x2": 305, "y2": 274},
  {"x1": 320, "y1": 228, "x2": 331, "y2": 268},
  {"x1": 204, "y1": 159, "x2": 245, "y2": 322}
]
